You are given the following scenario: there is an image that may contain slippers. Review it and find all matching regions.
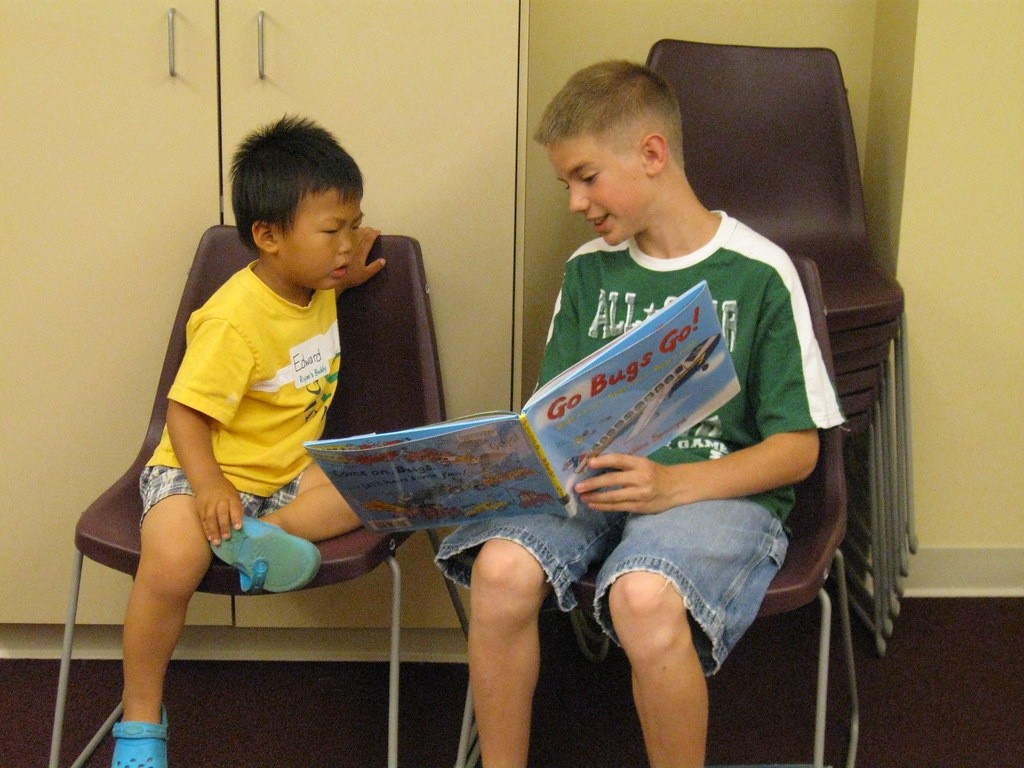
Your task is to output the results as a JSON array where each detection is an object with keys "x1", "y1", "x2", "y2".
[
  {"x1": 111, "y1": 703, "x2": 168, "y2": 768},
  {"x1": 210, "y1": 517, "x2": 322, "y2": 593}
]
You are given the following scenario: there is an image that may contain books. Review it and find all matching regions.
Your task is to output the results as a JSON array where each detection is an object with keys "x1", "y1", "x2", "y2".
[{"x1": 303, "y1": 280, "x2": 741, "y2": 534}]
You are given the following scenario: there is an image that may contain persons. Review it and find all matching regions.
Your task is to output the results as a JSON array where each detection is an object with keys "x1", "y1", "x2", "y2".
[
  {"x1": 434, "y1": 60, "x2": 847, "y2": 768},
  {"x1": 111, "y1": 117, "x2": 386, "y2": 768}
]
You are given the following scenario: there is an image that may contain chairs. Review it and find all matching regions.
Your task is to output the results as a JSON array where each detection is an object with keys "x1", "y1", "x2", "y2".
[{"x1": 44, "y1": 39, "x2": 917, "y2": 768}]
